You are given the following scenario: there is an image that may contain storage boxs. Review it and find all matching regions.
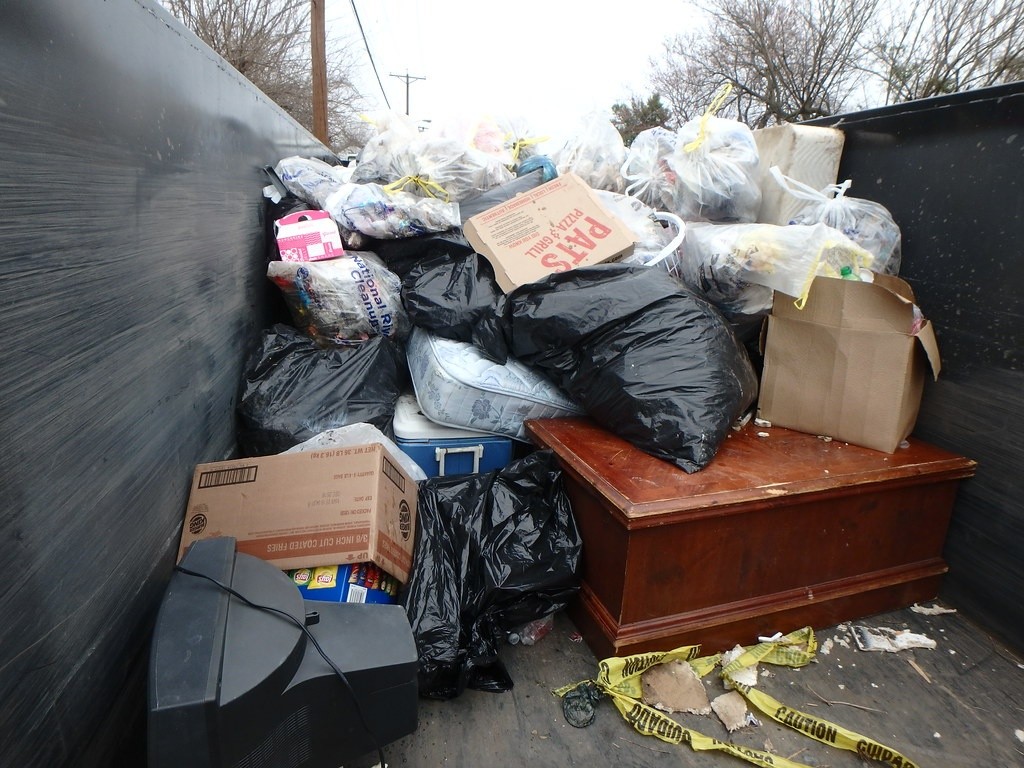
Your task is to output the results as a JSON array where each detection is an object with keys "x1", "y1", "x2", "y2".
[
  {"x1": 392, "y1": 394, "x2": 515, "y2": 477},
  {"x1": 283, "y1": 562, "x2": 402, "y2": 605},
  {"x1": 176, "y1": 441, "x2": 420, "y2": 585},
  {"x1": 463, "y1": 171, "x2": 636, "y2": 295},
  {"x1": 757, "y1": 264, "x2": 941, "y2": 454},
  {"x1": 273, "y1": 210, "x2": 343, "y2": 262}
]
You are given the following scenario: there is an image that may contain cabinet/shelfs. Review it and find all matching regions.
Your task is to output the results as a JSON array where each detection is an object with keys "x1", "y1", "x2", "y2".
[{"x1": 523, "y1": 416, "x2": 978, "y2": 665}]
